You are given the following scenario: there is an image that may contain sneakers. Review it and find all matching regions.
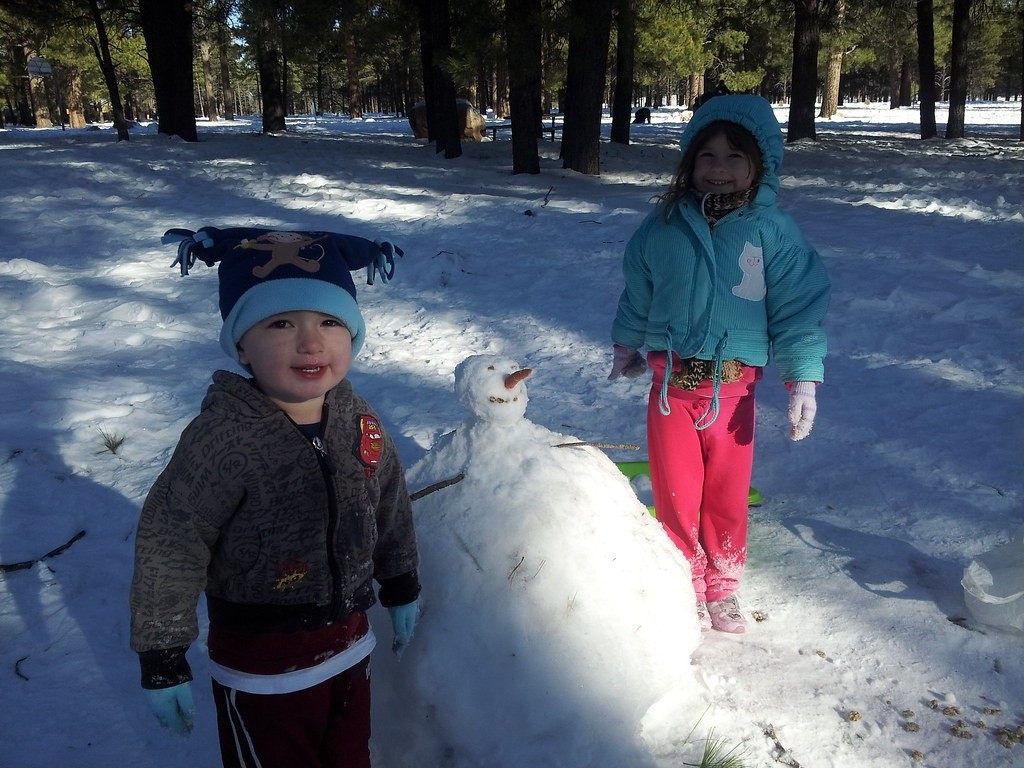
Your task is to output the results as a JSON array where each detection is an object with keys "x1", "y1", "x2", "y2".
[{"x1": 697, "y1": 593, "x2": 748, "y2": 633}]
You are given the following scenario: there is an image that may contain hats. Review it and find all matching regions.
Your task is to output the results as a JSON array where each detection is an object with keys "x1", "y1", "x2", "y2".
[{"x1": 161, "y1": 226, "x2": 404, "y2": 377}]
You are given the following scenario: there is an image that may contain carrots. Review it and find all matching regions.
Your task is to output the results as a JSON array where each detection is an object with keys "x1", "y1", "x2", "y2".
[{"x1": 505, "y1": 369, "x2": 532, "y2": 390}]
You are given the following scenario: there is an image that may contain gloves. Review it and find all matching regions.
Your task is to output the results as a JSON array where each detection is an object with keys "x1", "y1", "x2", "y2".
[
  {"x1": 144, "y1": 682, "x2": 196, "y2": 739},
  {"x1": 788, "y1": 382, "x2": 816, "y2": 441},
  {"x1": 389, "y1": 597, "x2": 420, "y2": 658},
  {"x1": 608, "y1": 344, "x2": 647, "y2": 380}
]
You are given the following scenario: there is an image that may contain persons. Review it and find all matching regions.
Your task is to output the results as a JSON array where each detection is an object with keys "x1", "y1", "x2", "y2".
[
  {"x1": 127, "y1": 231, "x2": 422, "y2": 768},
  {"x1": 606, "y1": 95, "x2": 832, "y2": 635}
]
